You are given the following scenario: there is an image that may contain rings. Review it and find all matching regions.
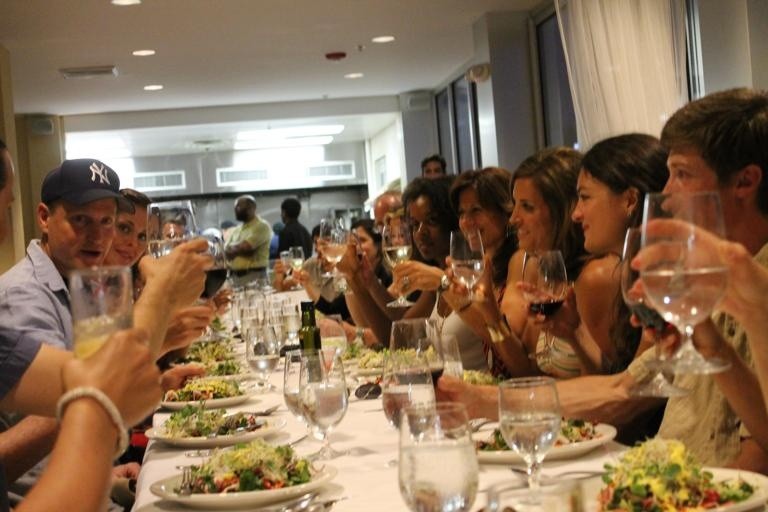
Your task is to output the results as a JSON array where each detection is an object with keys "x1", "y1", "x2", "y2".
[{"x1": 404, "y1": 276, "x2": 409, "y2": 288}]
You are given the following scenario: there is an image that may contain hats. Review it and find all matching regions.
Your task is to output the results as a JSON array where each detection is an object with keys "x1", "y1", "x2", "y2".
[{"x1": 41, "y1": 158, "x2": 136, "y2": 215}]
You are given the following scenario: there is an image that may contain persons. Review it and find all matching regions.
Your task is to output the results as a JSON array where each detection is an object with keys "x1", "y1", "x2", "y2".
[
  {"x1": 269, "y1": 222, "x2": 282, "y2": 258},
  {"x1": 224, "y1": 194, "x2": 271, "y2": 282},
  {"x1": 292, "y1": 219, "x2": 393, "y2": 322},
  {"x1": 273, "y1": 223, "x2": 355, "y2": 328},
  {"x1": 279, "y1": 198, "x2": 313, "y2": 261},
  {"x1": 421, "y1": 154, "x2": 446, "y2": 179},
  {"x1": 446, "y1": 147, "x2": 582, "y2": 378},
  {"x1": 316, "y1": 174, "x2": 457, "y2": 350},
  {"x1": 382, "y1": 86, "x2": 768, "y2": 476},
  {"x1": 0, "y1": 325, "x2": 164, "y2": 512},
  {"x1": 104, "y1": 188, "x2": 151, "y2": 268},
  {"x1": 146, "y1": 205, "x2": 161, "y2": 254},
  {"x1": 318, "y1": 205, "x2": 410, "y2": 329},
  {"x1": 517, "y1": 134, "x2": 666, "y2": 374},
  {"x1": 386, "y1": 166, "x2": 511, "y2": 375},
  {"x1": 160, "y1": 220, "x2": 183, "y2": 250}
]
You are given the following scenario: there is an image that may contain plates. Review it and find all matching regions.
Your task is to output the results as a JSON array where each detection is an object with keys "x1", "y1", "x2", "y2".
[
  {"x1": 579, "y1": 466, "x2": 768, "y2": 512},
  {"x1": 150, "y1": 462, "x2": 338, "y2": 512},
  {"x1": 144, "y1": 411, "x2": 287, "y2": 448},
  {"x1": 457, "y1": 421, "x2": 617, "y2": 465},
  {"x1": 161, "y1": 394, "x2": 249, "y2": 410}
]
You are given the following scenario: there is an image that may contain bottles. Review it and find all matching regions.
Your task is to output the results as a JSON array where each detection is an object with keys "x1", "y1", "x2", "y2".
[{"x1": 298, "y1": 300, "x2": 322, "y2": 383}]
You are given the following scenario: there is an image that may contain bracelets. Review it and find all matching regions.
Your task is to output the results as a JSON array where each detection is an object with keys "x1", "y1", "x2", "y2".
[
  {"x1": 57, "y1": 387, "x2": 129, "y2": 463},
  {"x1": 455, "y1": 300, "x2": 472, "y2": 315},
  {"x1": 486, "y1": 313, "x2": 512, "y2": 344}
]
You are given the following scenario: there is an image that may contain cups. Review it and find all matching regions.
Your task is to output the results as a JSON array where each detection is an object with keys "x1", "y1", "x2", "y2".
[
  {"x1": 241, "y1": 307, "x2": 258, "y2": 341},
  {"x1": 619, "y1": 228, "x2": 692, "y2": 398},
  {"x1": 297, "y1": 351, "x2": 349, "y2": 461},
  {"x1": 449, "y1": 229, "x2": 485, "y2": 304},
  {"x1": 70, "y1": 265, "x2": 133, "y2": 358},
  {"x1": 381, "y1": 346, "x2": 437, "y2": 442},
  {"x1": 280, "y1": 250, "x2": 292, "y2": 276},
  {"x1": 397, "y1": 402, "x2": 480, "y2": 512},
  {"x1": 521, "y1": 252, "x2": 568, "y2": 363},
  {"x1": 389, "y1": 317, "x2": 443, "y2": 386},
  {"x1": 320, "y1": 216, "x2": 352, "y2": 295},
  {"x1": 145, "y1": 199, "x2": 196, "y2": 258},
  {"x1": 382, "y1": 221, "x2": 416, "y2": 307},
  {"x1": 498, "y1": 376, "x2": 562, "y2": 512},
  {"x1": 246, "y1": 326, "x2": 281, "y2": 391},
  {"x1": 289, "y1": 247, "x2": 305, "y2": 291},
  {"x1": 417, "y1": 336, "x2": 464, "y2": 382},
  {"x1": 281, "y1": 305, "x2": 301, "y2": 346},
  {"x1": 639, "y1": 191, "x2": 731, "y2": 377},
  {"x1": 193, "y1": 234, "x2": 229, "y2": 344},
  {"x1": 281, "y1": 349, "x2": 326, "y2": 442}
]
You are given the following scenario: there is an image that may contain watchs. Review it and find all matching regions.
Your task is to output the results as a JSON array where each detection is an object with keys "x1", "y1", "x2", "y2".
[{"x1": 441, "y1": 274, "x2": 449, "y2": 290}]
